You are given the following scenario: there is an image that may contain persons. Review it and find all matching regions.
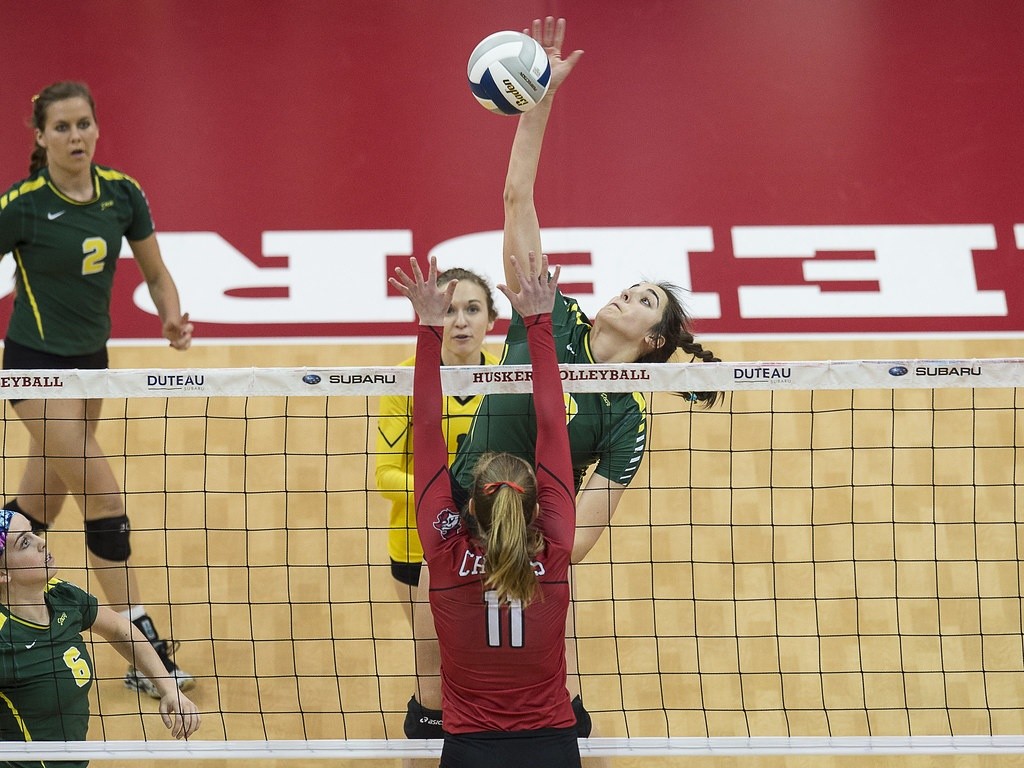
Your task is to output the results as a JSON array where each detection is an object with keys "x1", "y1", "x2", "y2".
[
  {"x1": 401, "y1": 16, "x2": 727, "y2": 768},
  {"x1": 0, "y1": 80, "x2": 196, "y2": 700},
  {"x1": 0, "y1": 507, "x2": 201, "y2": 768},
  {"x1": 384, "y1": 250, "x2": 583, "y2": 768},
  {"x1": 369, "y1": 267, "x2": 500, "y2": 629}
]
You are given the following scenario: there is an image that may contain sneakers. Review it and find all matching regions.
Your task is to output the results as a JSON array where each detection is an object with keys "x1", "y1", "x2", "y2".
[{"x1": 124, "y1": 614, "x2": 196, "y2": 699}]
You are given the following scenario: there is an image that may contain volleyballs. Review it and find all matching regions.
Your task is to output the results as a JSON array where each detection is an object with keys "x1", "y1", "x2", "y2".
[{"x1": 464, "y1": 30, "x2": 552, "y2": 117}]
